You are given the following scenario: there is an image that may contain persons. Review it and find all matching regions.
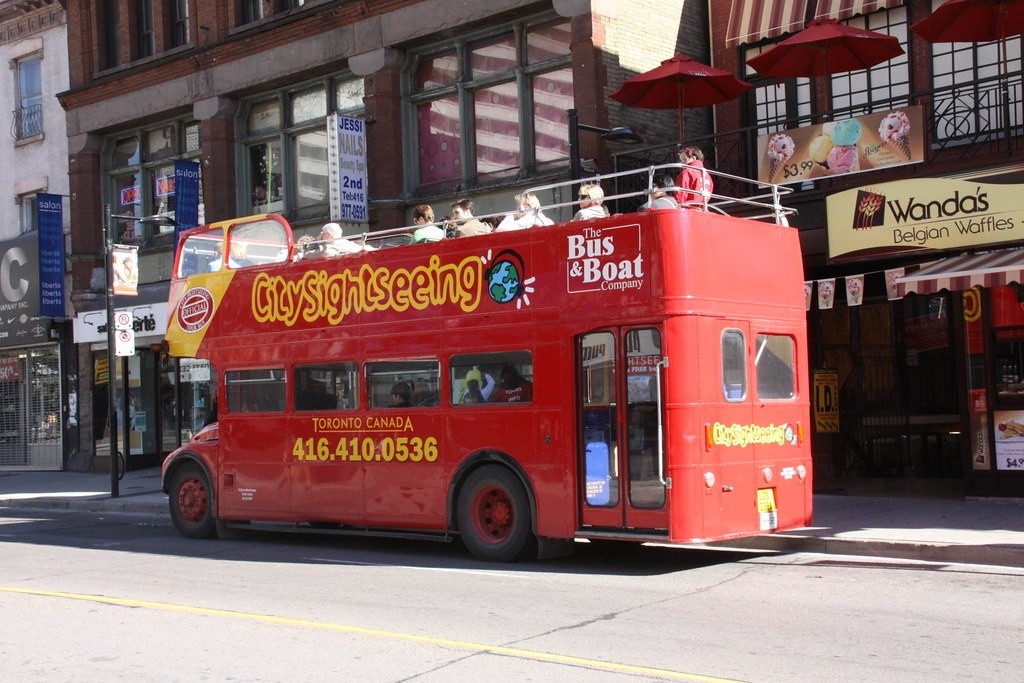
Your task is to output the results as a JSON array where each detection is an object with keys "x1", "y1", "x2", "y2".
[
  {"x1": 292, "y1": 236, "x2": 319, "y2": 263},
  {"x1": 390, "y1": 381, "x2": 414, "y2": 407},
  {"x1": 478, "y1": 216, "x2": 504, "y2": 232},
  {"x1": 304, "y1": 222, "x2": 361, "y2": 260},
  {"x1": 493, "y1": 362, "x2": 532, "y2": 402},
  {"x1": 448, "y1": 198, "x2": 491, "y2": 238},
  {"x1": 674, "y1": 144, "x2": 714, "y2": 213},
  {"x1": 637, "y1": 172, "x2": 679, "y2": 210},
  {"x1": 464, "y1": 368, "x2": 496, "y2": 402},
  {"x1": 570, "y1": 182, "x2": 611, "y2": 222},
  {"x1": 495, "y1": 191, "x2": 554, "y2": 232},
  {"x1": 408, "y1": 203, "x2": 445, "y2": 246},
  {"x1": 437, "y1": 214, "x2": 459, "y2": 239},
  {"x1": 229, "y1": 242, "x2": 253, "y2": 268},
  {"x1": 206, "y1": 241, "x2": 243, "y2": 272}
]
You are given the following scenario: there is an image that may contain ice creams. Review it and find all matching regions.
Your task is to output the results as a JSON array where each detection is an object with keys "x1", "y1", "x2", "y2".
[{"x1": 767, "y1": 110, "x2": 912, "y2": 184}]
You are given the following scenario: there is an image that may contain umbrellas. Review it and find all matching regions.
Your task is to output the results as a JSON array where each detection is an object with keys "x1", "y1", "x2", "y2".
[
  {"x1": 747, "y1": 16, "x2": 906, "y2": 116},
  {"x1": 606, "y1": 53, "x2": 754, "y2": 148},
  {"x1": 908, "y1": 0, "x2": 1023, "y2": 101}
]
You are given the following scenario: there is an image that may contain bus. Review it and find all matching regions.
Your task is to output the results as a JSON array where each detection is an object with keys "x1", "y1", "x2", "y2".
[{"x1": 156, "y1": 161, "x2": 819, "y2": 576}]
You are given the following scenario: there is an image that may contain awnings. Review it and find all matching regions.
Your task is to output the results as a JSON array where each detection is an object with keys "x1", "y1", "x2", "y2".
[
  {"x1": 894, "y1": 248, "x2": 1023, "y2": 300},
  {"x1": 725, "y1": 0, "x2": 902, "y2": 49}
]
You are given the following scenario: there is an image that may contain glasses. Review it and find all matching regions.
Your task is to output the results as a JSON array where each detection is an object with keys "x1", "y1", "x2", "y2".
[
  {"x1": 680, "y1": 157, "x2": 689, "y2": 162},
  {"x1": 320, "y1": 232, "x2": 330, "y2": 236},
  {"x1": 578, "y1": 195, "x2": 590, "y2": 200}
]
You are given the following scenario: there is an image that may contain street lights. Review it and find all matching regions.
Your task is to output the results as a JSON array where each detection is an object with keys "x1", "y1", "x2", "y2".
[
  {"x1": 564, "y1": 105, "x2": 646, "y2": 222},
  {"x1": 96, "y1": 202, "x2": 179, "y2": 496}
]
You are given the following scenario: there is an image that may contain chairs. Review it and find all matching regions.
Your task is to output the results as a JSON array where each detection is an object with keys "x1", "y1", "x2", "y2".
[{"x1": 417, "y1": 401, "x2": 432, "y2": 408}]
